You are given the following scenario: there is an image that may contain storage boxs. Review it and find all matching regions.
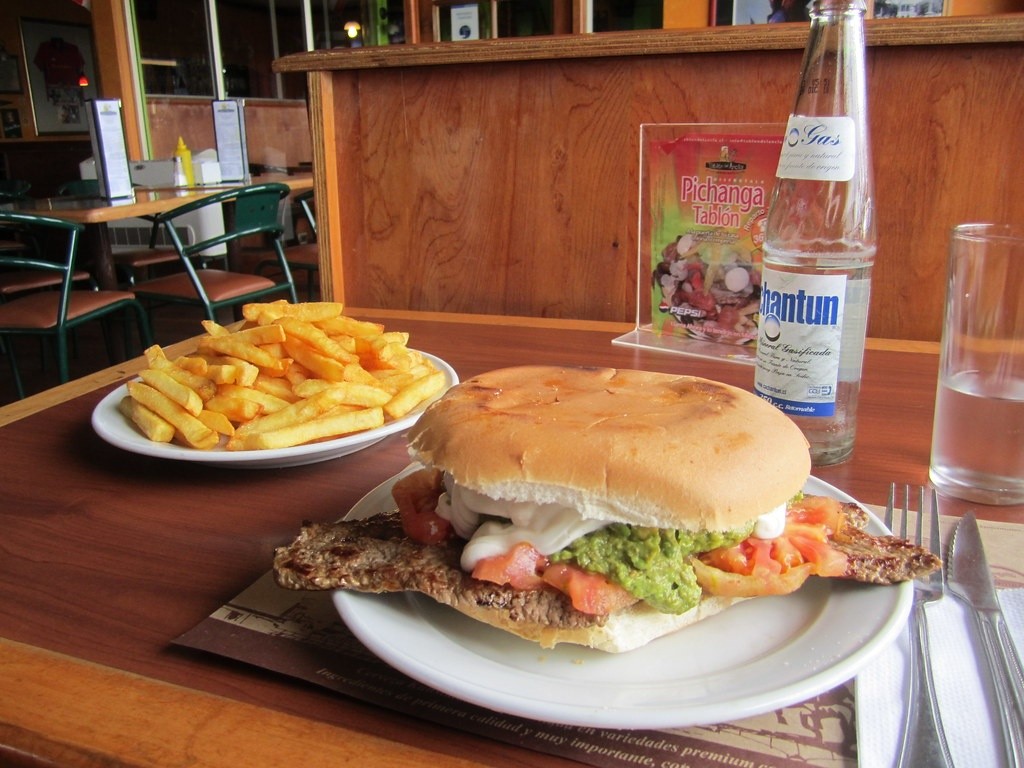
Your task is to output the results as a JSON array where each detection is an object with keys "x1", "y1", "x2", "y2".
[{"x1": 0, "y1": 307, "x2": 1024, "y2": 768}]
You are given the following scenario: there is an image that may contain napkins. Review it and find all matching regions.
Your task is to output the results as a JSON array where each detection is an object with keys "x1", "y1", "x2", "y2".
[{"x1": 853, "y1": 586, "x2": 1024, "y2": 768}]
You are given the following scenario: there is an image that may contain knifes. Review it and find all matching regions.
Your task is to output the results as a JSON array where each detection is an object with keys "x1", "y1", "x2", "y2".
[{"x1": 948, "y1": 509, "x2": 1024, "y2": 768}]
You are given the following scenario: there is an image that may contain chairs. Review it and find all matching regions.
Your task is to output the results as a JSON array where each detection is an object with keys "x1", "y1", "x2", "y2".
[
  {"x1": 124, "y1": 183, "x2": 299, "y2": 362},
  {"x1": 0, "y1": 211, "x2": 153, "y2": 399},
  {"x1": 253, "y1": 189, "x2": 319, "y2": 303}
]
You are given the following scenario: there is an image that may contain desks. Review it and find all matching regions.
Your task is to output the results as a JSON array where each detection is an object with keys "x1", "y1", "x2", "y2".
[
  {"x1": 0, "y1": 188, "x2": 235, "y2": 366},
  {"x1": 190, "y1": 171, "x2": 314, "y2": 322}
]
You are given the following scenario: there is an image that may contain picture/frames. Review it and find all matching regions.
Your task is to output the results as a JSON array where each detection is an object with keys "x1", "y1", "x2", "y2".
[
  {"x1": 17, "y1": 15, "x2": 100, "y2": 137},
  {"x1": 0, "y1": 55, "x2": 25, "y2": 95}
]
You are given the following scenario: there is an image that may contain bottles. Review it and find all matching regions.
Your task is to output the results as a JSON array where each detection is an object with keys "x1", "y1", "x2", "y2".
[
  {"x1": 173, "y1": 157, "x2": 188, "y2": 197},
  {"x1": 174, "y1": 136, "x2": 196, "y2": 188},
  {"x1": 754, "y1": 0, "x2": 880, "y2": 467}
]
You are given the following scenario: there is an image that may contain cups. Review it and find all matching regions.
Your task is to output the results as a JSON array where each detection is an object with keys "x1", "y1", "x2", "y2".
[{"x1": 928, "y1": 222, "x2": 1024, "y2": 504}]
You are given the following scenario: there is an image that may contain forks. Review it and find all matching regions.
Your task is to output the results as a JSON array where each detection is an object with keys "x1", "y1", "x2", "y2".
[{"x1": 880, "y1": 480, "x2": 954, "y2": 768}]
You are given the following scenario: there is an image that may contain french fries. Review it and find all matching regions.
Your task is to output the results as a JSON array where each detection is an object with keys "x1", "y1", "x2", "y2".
[{"x1": 118, "y1": 299, "x2": 444, "y2": 450}]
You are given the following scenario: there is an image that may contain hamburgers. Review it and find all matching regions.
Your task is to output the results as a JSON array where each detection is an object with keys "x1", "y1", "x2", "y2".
[{"x1": 392, "y1": 366, "x2": 834, "y2": 653}]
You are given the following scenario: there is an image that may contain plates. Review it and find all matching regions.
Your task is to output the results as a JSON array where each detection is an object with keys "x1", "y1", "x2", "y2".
[
  {"x1": 334, "y1": 472, "x2": 918, "y2": 729},
  {"x1": 91, "y1": 347, "x2": 461, "y2": 466}
]
